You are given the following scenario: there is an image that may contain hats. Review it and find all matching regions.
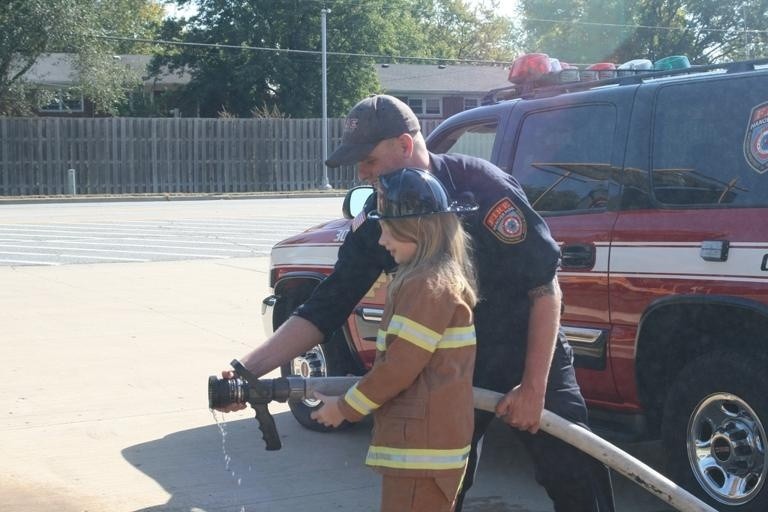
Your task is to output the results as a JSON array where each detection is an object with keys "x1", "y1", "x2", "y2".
[{"x1": 324, "y1": 93, "x2": 422, "y2": 169}]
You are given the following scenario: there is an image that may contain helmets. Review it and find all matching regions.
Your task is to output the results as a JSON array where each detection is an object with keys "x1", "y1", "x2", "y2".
[{"x1": 365, "y1": 168, "x2": 479, "y2": 219}]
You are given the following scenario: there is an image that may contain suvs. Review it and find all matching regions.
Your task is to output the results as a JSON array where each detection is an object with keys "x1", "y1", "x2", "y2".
[{"x1": 262, "y1": 53, "x2": 768, "y2": 512}]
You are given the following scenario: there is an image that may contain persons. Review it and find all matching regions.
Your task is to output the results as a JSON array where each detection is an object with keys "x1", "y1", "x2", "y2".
[
  {"x1": 310, "y1": 167, "x2": 479, "y2": 512},
  {"x1": 216, "y1": 94, "x2": 616, "y2": 512}
]
